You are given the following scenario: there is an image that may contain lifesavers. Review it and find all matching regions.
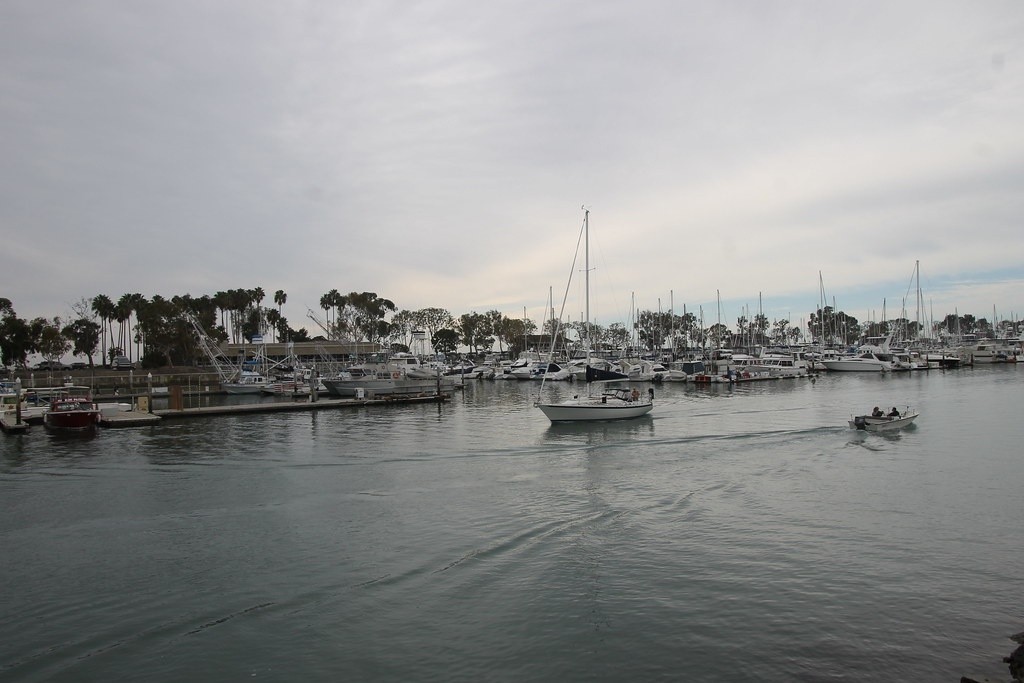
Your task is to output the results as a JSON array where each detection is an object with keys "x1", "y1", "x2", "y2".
[{"x1": 392, "y1": 372, "x2": 399, "y2": 378}]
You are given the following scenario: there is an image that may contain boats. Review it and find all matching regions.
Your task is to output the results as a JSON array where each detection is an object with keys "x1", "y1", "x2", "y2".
[
  {"x1": 41, "y1": 382, "x2": 102, "y2": 441},
  {"x1": 545, "y1": 415, "x2": 655, "y2": 441},
  {"x1": 847, "y1": 408, "x2": 922, "y2": 432}
]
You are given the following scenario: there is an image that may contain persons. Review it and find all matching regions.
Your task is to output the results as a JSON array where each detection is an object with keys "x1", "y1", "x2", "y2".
[
  {"x1": 887, "y1": 407, "x2": 900, "y2": 418},
  {"x1": 872, "y1": 406, "x2": 883, "y2": 417}
]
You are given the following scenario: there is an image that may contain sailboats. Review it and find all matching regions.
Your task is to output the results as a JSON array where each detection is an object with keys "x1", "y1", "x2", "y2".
[
  {"x1": 217, "y1": 259, "x2": 1024, "y2": 398},
  {"x1": 529, "y1": 206, "x2": 657, "y2": 420}
]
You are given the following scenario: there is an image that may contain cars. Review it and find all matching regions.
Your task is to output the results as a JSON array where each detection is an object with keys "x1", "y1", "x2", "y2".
[
  {"x1": 9, "y1": 363, "x2": 27, "y2": 372},
  {"x1": 111, "y1": 356, "x2": 134, "y2": 372},
  {"x1": 70, "y1": 362, "x2": 88, "y2": 370},
  {"x1": 33, "y1": 361, "x2": 75, "y2": 372}
]
